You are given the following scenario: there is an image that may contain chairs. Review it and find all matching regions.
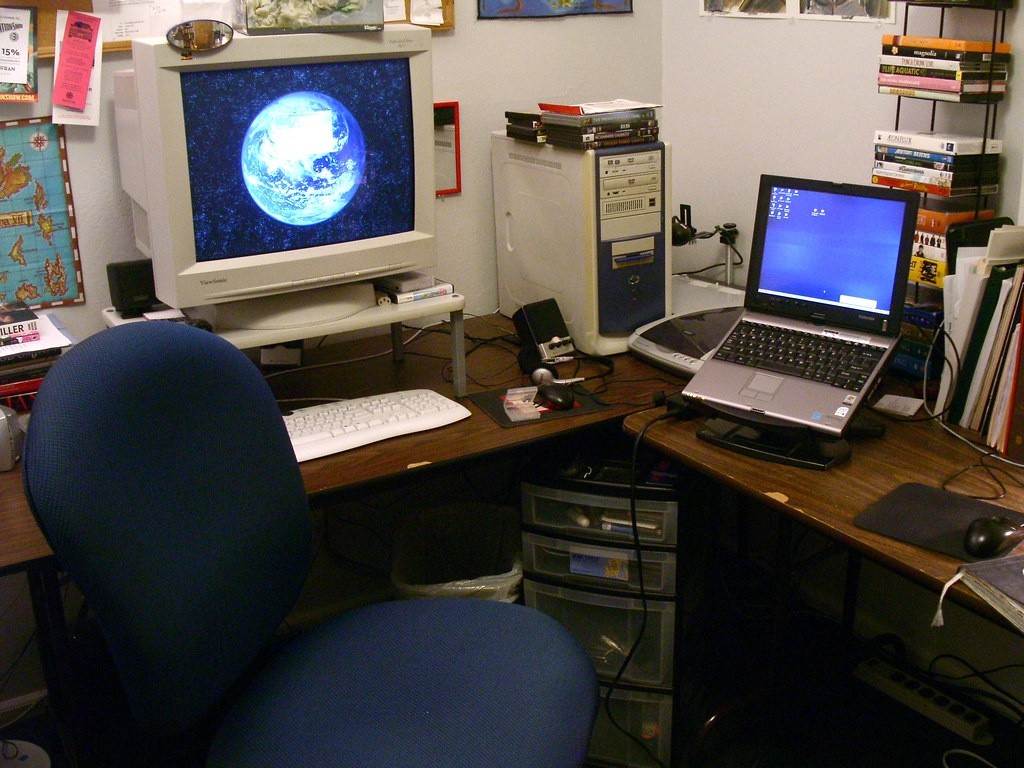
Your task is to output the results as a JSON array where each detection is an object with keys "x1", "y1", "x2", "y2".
[{"x1": 23, "y1": 320, "x2": 600, "y2": 768}]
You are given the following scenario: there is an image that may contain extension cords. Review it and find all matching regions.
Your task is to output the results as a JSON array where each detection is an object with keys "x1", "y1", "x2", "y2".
[{"x1": 853, "y1": 656, "x2": 994, "y2": 746}]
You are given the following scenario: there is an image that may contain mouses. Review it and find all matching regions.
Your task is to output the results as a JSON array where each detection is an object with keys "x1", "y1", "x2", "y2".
[
  {"x1": 534, "y1": 384, "x2": 574, "y2": 411},
  {"x1": 963, "y1": 515, "x2": 1024, "y2": 560}
]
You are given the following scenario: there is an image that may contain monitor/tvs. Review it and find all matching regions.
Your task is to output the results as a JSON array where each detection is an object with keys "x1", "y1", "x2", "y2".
[{"x1": 111, "y1": 23, "x2": 437, "y2": 332}]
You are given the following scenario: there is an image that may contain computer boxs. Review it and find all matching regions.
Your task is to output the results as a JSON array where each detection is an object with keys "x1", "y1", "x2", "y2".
[{"x1": 492, "y1": 136, "x2": 672, "y2": 357}]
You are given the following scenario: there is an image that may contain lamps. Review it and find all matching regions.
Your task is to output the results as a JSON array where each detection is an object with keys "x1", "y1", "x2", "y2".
[{"x1": 673, "y1": 204, "x2": 739, "y2": 284}]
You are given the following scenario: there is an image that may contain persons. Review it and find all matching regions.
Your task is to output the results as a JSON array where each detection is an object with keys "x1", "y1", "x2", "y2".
[
  {"x1": 925, "y1": 235, "x2": 929, "y2": 245},
  {"x1": 930, "y1": 235, "x2": 935, "y2": 246},
  {"x1": 937, "y1": 236, "x2": 941, "y2": 248},
  {"x1": 921, "y1": 234, "x2": 924, "y2": 244},
  {"x1": 914, "y1": 245, "x2": 924, "y2": 258},
  {"x1": 915, "y1": 234, "x2": 919, "y2": 243}
]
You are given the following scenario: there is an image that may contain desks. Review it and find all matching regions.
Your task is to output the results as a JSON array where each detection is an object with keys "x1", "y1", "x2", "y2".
[
  {"x1": 623, "y1": 376, "x2": 1023, "y2": 636},
  {"x1": 0, "y1": 275, "x2": 690, "y2": 768}
]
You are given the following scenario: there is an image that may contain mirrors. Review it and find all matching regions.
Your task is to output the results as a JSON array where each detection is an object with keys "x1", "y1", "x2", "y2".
[{"x1": 432, "y1": 101, "x2": 462, "y2": 195}]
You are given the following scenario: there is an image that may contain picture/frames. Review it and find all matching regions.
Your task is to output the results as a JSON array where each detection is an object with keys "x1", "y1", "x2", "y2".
[{"x1": 0, "y1": 116, "x2": 86, "y2": 311}]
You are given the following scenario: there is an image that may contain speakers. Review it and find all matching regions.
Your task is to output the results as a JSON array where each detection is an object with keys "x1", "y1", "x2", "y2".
[
  {"x1": 512, "y1": 299, "x2": 577, "y2": 364},
  {"x1": 106, "y1": 259, "x2": 157, "y2": 319}
]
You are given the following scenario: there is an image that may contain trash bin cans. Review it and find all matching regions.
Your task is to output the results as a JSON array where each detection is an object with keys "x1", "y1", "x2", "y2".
[{"x1": 382, "y1": 543, "x2": 524, "y2": 607}]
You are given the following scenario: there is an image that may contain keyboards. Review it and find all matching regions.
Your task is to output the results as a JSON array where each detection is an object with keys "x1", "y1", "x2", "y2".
[{"x1": 281, "y1": 389, "x2": 472, "y2": 463}]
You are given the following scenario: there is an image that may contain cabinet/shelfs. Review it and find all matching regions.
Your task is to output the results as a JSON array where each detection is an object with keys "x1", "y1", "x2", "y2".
[
  {"x1": 519, "y1": 439, "x2": 705, "y2": 767},
  {"x1": 887, "y1": 1, "x2": 1017, "y2": 382}
]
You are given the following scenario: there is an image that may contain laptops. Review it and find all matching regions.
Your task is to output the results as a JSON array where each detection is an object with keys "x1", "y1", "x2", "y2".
[{"x1": 683, "y1": 174, "x2": 920, "y2": 438}]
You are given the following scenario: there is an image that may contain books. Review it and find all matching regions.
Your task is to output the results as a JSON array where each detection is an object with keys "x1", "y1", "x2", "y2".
[
  {"x1": 892, "y1": 289, "x2": 944, "y2": 380},
  {"x1": 503, "y1": 100, "x2": 659, "y2": 149},
  {"x1": 877, "y1": 35, "x2": 1011, "y2": 104},
  {"x1": 0, "y1": 298, "x2": 76, "y2": 420},
  {"x1": 872, "y1": 130, "x2": 1003, "y2": 197},
  {"x1": 907, "y1": 209, "x2": 996, "y2": 289},
  {"x1": 931, "y1": 226, "x2": 1024, "y2": 465},
  {"x1": 930, "y1": 555, "x2": 1024, "y2": 639}
]
